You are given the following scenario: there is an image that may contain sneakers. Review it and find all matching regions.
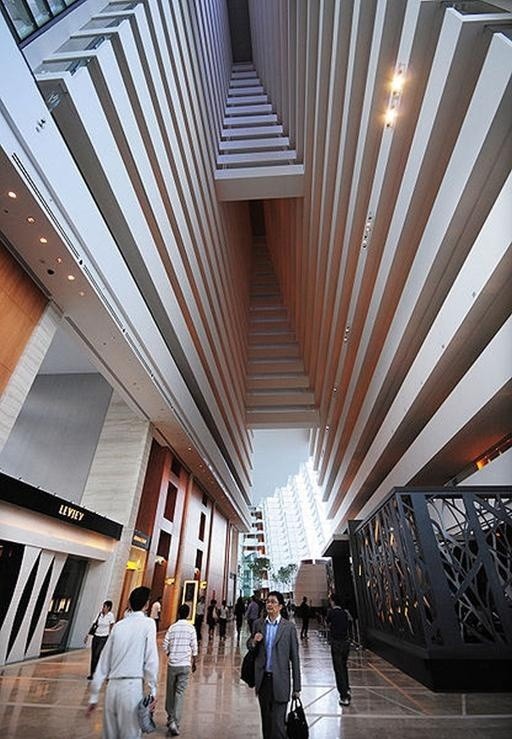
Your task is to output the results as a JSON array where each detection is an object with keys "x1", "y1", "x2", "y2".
[
  {"x1": 338, "y1": 687, "x2": 353, "y2": 708},
  {"x1": 166, "y1": 722, "x2": 180, "y2": 736}
]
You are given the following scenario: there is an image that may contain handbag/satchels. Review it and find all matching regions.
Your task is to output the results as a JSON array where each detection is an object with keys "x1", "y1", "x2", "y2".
[
  {"x1": 284, "y1": 696, "x2": 311, "y2": 739},
  {"x1": 88, "y1": 622, "x2": 99, "y2": 636},
  {"x1": 238, "y1": 650, "x2": 256, "y2": 690}
]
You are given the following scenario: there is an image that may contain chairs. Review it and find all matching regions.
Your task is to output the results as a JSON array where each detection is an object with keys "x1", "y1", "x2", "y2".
[{"x1": 41, "y1": 619, "x2": 68, "y2": 649}]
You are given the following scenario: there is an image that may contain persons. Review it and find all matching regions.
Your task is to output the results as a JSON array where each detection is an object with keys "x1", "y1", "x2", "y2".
[
  {"x1": 324, "y1": 592, "x2": 352, "y2": 706},
  {"x1": 193, "y1": 596, "x2": 207, "y2": 641},
  {"x1": 218, "y1": 600, "x2": 230, "y2": 642},
  {"x1": 202, "y1": 640, "x2": 215, "y2": 684},
  {"x1": 214, "y1": 640, "x2": 226, "y2": 679},
  {"x1": 206, "y1": 597, "x2": 219, "y2": 641},
  {"x1": 83, "y1": 600, "x2": 115, "y2": 681},
  {"x1": 85, "y1": 585, "x2": 161, "y2": 738},
  {"x1": 150, "y1": 595, "x2": 163, "y2": 632},
  {"x1": 234, "y1": 596, "x2": 245, "y2": 640},
  {"x1": 230, "y1": 640, "x2": 242, "y2": 685},
  {"x1": 243, "y1": 594, "x2": 297, "y2": 635},
  {"x1": 191, "y1": 642, "x2": 202, "y2": 684},
  {"x1": 247, "y1": 590, "x2": 303, "y2": 739},
  {"x1": 298, "y1": 596, "x2": 311, "y2": 640},
  {"x1": 163, "y1": 604, "x2": 199, "y2": 736}
]
